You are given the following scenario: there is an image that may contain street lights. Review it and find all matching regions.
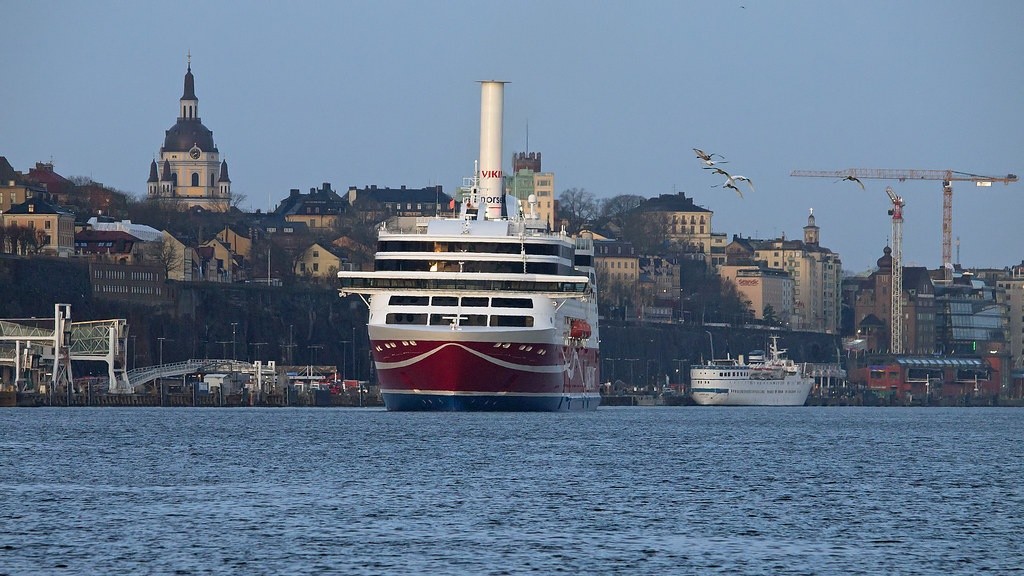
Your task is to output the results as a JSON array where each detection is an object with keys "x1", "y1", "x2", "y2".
[
  {"x1": 339, "y1": 340, "x2": 352, "y2": 380},
  {"x1": 289, "y1": 324, "x2": 293, "y2": 366},
  {"x1": 131, "y1": 335, "x2": 137, "y2": 369},
  {"x1": 646, "y1": 358, "x2": 656, "y2": 391},
  {"x1": 280, "y1": 344, "x2": 298, "y2": 362},
  {"x1": 230, "y1": 323, "x2": 239, "y2": 360},
  {"x1": 308, "y1": 345, "x2": 323, "y2": 366},
  {"x1": 705, "y1": 330, "x2": 715, "y2": 365},
  {"x1": 623, "y1": 358, "x2": 640, "y2": 387},
  {"x1": 249, "y1": 343, "x2": 269, "y2": 361},
  {"x1": 353, "y1": 327, "x2": 357, "y2": 380},
  {"x1": 606, "y1": 357, "x2": 622, "y2": 391},
  {"x1": 157, "y1": 337, "x2": 166, "y2": 389},
  {"x1": 673, "y1": 358, "x2": 688, "y2": 394},
  {"x1": 215, "y1": 340, "x2": 234, "y2": 360}
]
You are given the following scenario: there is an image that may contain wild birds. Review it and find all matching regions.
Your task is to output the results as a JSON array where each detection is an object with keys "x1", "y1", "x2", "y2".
[
  {"x1": 726, "y1": 175, "x2": 755, "y2": 192},
  {"x1": 691, "y1": 148, "x2": 724, "y2": 160},
  {"x1": 843, "y1": 176, "x2": 864, "y2": 189},
  {"x1": 700, "y1": 160, "x2": 729, "y2": 167},
  {"x1": 703, "y1": 167, "x2": 738, "y2": 184},
  {"x1": 711, "y1": 184, "x2": 745, "y2": 199}
]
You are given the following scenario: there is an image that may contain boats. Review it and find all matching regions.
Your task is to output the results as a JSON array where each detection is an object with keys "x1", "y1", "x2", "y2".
[
  {"x1": 688, "y1": 334, "x2": 816, "y2": 407},
  {"x1": 571, "y1": 321, "x2": 592, "y2": 339}
]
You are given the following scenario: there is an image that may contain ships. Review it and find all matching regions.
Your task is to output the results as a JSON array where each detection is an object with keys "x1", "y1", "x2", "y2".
[{"x1": 337, "y1": 80, "x2": 605, "y2": 413}]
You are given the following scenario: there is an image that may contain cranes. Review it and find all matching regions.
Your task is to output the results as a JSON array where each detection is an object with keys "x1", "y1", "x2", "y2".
[
  {"x1": 885, "y1": 186, "x2": 909, "y2": 357},
  {"x1": 790, "y1": 166, "x2": 1020, "y2": 355}
]
